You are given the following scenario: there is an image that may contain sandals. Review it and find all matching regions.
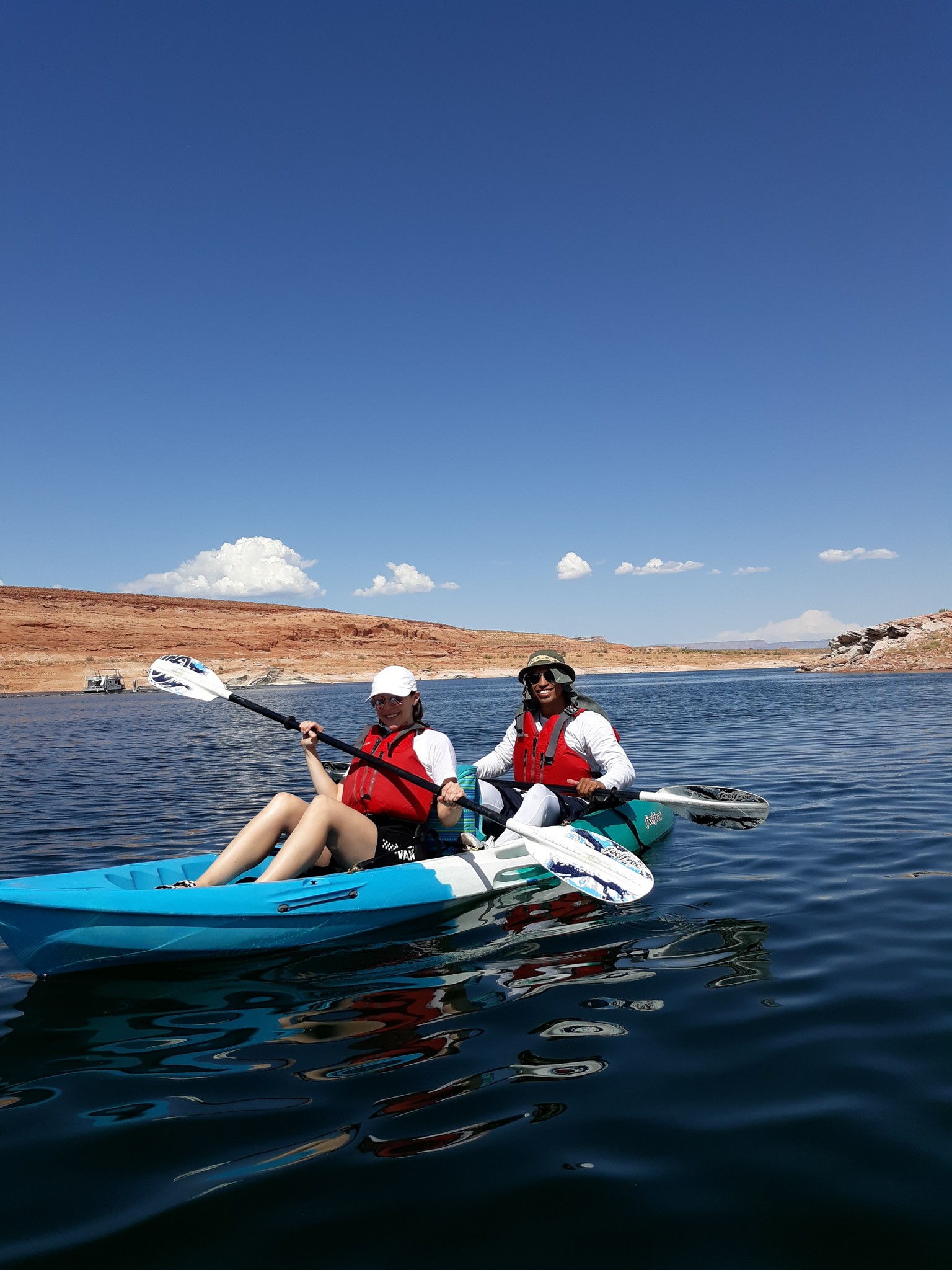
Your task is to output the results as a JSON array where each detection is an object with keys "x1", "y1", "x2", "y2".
[{"x1": 153, "y1": 880, "x2": 197, "y2": 889}]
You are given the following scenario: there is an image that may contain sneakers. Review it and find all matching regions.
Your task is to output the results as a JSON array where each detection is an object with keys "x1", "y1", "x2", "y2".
[{"x1": 456, "y1": 832, "x2": 495, "y2": 848}]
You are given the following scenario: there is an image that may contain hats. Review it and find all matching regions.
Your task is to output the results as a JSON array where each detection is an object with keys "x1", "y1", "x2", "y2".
[
  {"x1": 365, "y1": 666, "x2": 418, "y2": 703},
  {"x1": 518, "y1": 650, "x2": 576, "y2": 685}
]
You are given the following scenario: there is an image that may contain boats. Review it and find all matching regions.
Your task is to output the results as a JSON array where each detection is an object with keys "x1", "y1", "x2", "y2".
[
  {"x1": 82, "y1": 669, "x2": 126, "y2": 694},
  {"x1": 0, "y1": 799, "x2": 673, "y2": 979}
]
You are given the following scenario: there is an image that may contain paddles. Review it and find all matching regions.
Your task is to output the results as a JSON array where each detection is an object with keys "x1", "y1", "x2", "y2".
[
  {"x1": 318, "y1": 760, "x2": 771, "y2": 831},
  {"x1": 148, "y1": 653, "x2": 656, "y2": 905}
]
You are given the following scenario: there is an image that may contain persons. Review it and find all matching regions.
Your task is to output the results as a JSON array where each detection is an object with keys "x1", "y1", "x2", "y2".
[
  {"x1": 460, "y1": 649, "x2": 635, "y2": 851},
  {"x1": 155, "y1": 665, "x2": 465, "y2": 890}
]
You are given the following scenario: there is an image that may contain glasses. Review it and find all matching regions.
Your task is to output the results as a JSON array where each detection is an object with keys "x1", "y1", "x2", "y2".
[
  {"x1": 527, "y1": 671, "x2": 555, "y2": 684},
  {"x1": 372, "y1": 695, "x2": 403, "y2": 710}
]
